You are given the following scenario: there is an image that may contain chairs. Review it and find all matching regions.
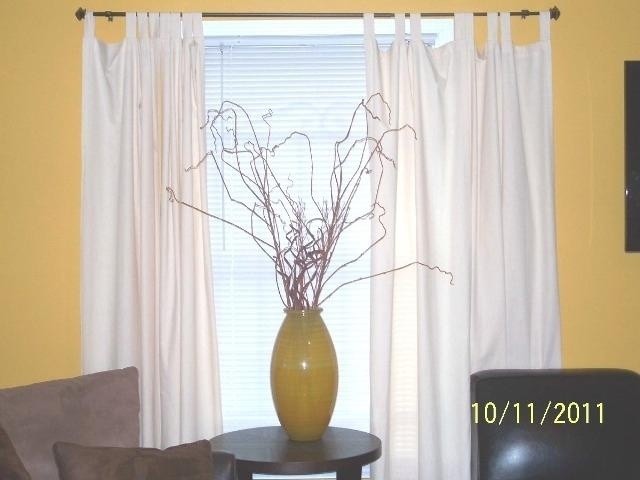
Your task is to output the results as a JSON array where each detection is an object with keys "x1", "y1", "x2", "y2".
[
  {"x1": 1, "y1": 365, "x2": 237, "y2": 479},
  {"x1": 471, "y1": 369, "x2": 640, "y2": 479}
]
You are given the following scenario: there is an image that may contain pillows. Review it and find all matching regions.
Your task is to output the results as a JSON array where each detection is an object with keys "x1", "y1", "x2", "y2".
[{"x1": 53, "y1": 438, "x2": 213, "y2": 479}]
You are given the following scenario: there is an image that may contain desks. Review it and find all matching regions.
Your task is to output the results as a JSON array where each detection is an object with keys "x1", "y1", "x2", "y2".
[{"x1": 210, "y1": 426, "x2": 383, "y2": 480}]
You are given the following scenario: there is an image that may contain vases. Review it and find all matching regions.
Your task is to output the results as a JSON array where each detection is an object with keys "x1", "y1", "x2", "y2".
[{"x1": 269, "y1": 308, "x2": 339, "y2": 443}]
